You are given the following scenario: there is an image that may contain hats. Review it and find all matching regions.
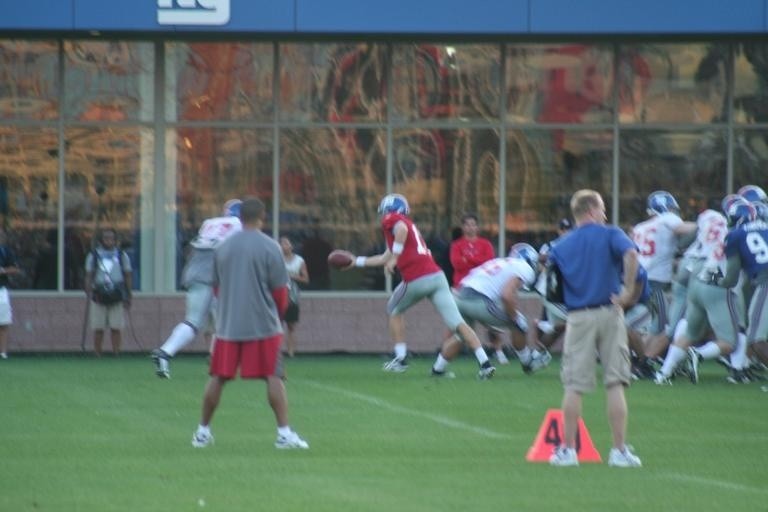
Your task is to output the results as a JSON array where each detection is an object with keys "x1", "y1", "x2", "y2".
[{"x1": 557, "y1": 216, "x2": 571, "y2": 229}]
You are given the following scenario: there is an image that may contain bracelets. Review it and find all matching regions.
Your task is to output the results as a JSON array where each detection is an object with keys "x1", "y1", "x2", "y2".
[
  {"x1": 390, "y1": 241, "x2": 405, "y2": 256},
  {"x1": 354, "y1": 256, "x2": 368, "y2": 268},
  {"x1": 0, "y1": 267, "x2": 5, "y2": 273}
]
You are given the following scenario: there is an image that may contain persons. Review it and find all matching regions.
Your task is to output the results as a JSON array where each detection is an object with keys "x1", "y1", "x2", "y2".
[
  {"x1": 336, "y1": 192, "x2": 498, "y2": 382},
  {"x1": 80, "y1": 227, "x2": 134, "y2": 360},
  {"x1": 148, "y1": 196, "x2": 244, "y2": 380},
  {"x1": 191, "y1": 194, "x2": 312, "y2": 452},
  {"x1": 424, "y1": 174, "x2": 767, "y2": 389},
  {"x1": 543, "y1": 184, "x2": 645, "y2": 471},
  {"x1": 275, "y1": 233, "x2": 311, "y2": 360},
  {"x1": 0, "y1": 227, "x2": 22, "y2": 359}
]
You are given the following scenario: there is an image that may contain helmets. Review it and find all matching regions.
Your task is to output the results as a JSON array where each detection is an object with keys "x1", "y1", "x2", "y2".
[
  {"x1": 646, "y1": 189, "x2": 679, "y2": 215},
  {"x1": 223, "y1": 199, "x2": 242, "y2": 217},
  {"x1": 719, "y1": 184, "x2": 767, "y2": 236},
  {"x1": 511, "y1": 243, "x2": 539, "y2": 271},
  {"x1": 377, "y1": 192, "x2": 409, "y2": 216}
]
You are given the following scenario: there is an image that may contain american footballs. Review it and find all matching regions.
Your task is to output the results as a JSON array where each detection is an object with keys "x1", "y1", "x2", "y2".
[{"x1": 328, "y1": 250, "x2": 353, "y2": 269}]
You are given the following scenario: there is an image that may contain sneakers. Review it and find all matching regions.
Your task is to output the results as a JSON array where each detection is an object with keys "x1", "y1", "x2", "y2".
[
  {"x1": 153, "y1": 350, "x2": 169, "y2": 380},
  {"x1": 550, "y1": 447, "x2": 579, "y2": 467},
  {"x1": 430, "y1": 365, "x2": 456, "y2": 379},
  {"x1": 608, "y1": 450, "x2": 642, "y2": 467},
  {"x1": 492, "y1": 350, "x2": 510, "y2": 365},
  {"x1": 273, "y1": 432, "x2": 310, "y2": 451},
  {"x1": 193, "y1": 430, "x2": 214, "y2": 448},
  {"x1": 474, "y1": 366, "x2": 496, "y2": 380},
  {"x1": 383, "y1": 358, "x2": 409, "y2": 372},
  {"x1": 522, "y1": 350, "x2": 554, "y2": 372},
  {"x1": 628, "y1": 342, "x2": 766, "y2": 388}
]
